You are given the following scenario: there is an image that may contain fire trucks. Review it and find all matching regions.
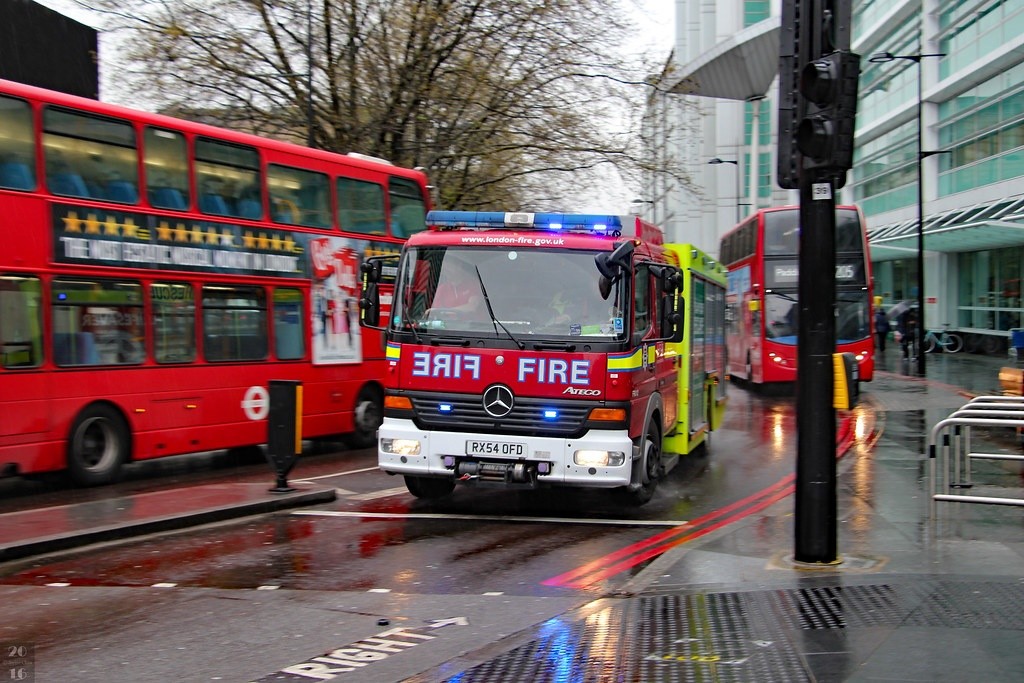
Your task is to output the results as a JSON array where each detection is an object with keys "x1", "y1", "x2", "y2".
[{"x1": 355, "y1": 207, "x2": 732, "y2": 505}]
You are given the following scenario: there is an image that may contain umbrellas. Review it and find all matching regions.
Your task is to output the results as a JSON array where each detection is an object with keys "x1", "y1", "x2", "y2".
[{"x1": 886, "y1": 298, "x2": 914, "y2": 319}]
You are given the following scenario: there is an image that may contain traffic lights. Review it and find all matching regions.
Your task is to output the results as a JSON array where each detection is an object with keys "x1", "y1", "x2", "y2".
[{"x1": 791, "y1": 50, "x2": 864, "y2": 172}]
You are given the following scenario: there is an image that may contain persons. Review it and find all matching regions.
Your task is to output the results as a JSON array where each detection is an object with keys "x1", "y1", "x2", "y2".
[
  {"x1": 319, "y1": 288, "x2": 353, "y2": 348},
  {"x1": 873, "y1": 304, "x2": 920, "y2": 363},
  {"x1": 547, "y1": 261, "x2": 650, "y2": 333},
  {"x1": 659, "y1": 376, "x2": 666, "y2": 392},
  {"x1": 416, "y1": 253, "x2": 490, "y2": 323},
  {"x1": 765, "y1": 306, "x2": 793, "y2": 337}
]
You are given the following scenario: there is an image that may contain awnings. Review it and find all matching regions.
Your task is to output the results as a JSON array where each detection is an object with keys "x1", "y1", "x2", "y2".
[{"x1": 864, "y1": 192, "x2": 1024, "y2": 262}]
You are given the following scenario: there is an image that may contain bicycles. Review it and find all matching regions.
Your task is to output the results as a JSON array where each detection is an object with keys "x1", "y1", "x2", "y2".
[{"x1": 923, "y1": 322, "x2": 964, "y2": 353}]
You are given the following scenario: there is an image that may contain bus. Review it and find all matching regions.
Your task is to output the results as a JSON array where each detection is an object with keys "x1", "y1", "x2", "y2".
[
  {"x1": 719, "y1": 202, "x2": 888, "y2": 394},
  {"x1": 0, "y1": 77, "x2": 438, "y2": 489}
]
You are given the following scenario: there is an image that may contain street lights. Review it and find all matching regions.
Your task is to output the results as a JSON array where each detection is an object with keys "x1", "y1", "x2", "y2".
[
  {"x1": 867, "y1": 48, "x2": 949, "y2": 378},
  {"x1": 633, "y1": 199, "x2": 657, "y2": 224},
  {"x1": 707, "y1": 155, "x2": 743, "y2": 224}
]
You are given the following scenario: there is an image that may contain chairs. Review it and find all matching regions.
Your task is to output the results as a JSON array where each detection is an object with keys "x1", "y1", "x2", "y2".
[
  {"x1": 39, "y1": 332, "x2": 103, "y2": 364},
  {"x1": 0, "y1": 152, "x2": 410, "y2": 237}
]
